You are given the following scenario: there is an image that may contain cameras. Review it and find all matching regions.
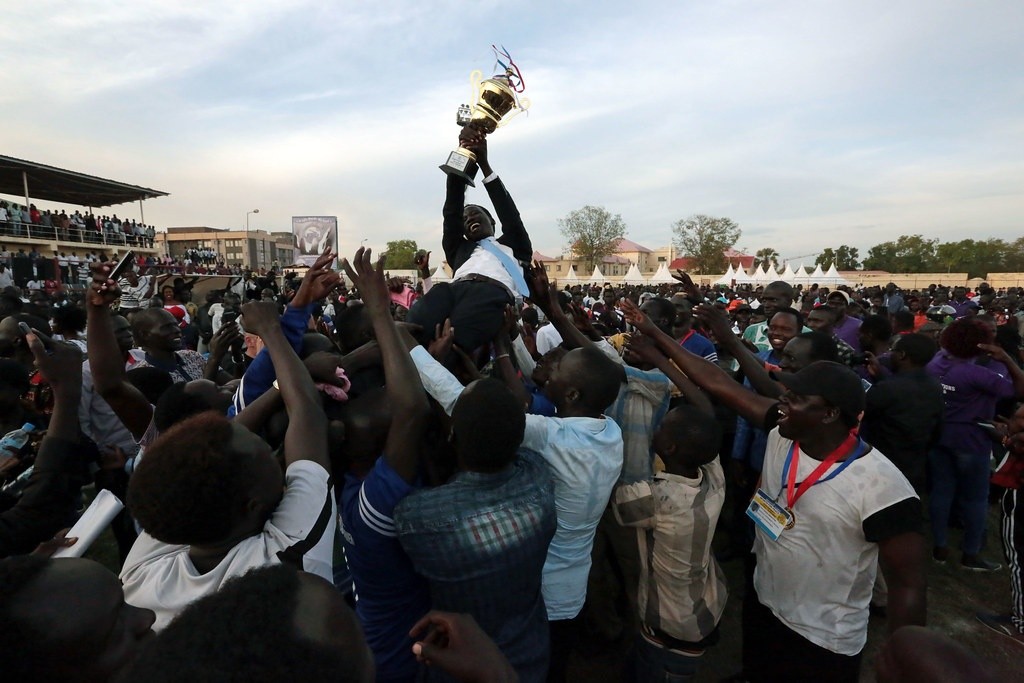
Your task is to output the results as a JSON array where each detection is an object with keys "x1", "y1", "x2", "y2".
[{"x1": 850, "y1": 352, "x2": 870, "y2": 366}]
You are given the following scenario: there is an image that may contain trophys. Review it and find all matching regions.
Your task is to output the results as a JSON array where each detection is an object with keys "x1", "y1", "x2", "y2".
[{"x1": 439, "y1": 46, "x2": 530, "y2": 188}]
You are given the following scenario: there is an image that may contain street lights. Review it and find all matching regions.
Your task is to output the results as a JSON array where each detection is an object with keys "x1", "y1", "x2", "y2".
[{"x1": 245, "y1": 209, "x2": 259, "y2": 231}]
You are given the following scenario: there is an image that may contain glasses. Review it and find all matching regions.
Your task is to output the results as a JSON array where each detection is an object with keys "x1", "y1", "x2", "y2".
[
  {"x1": 827, "y1": 300, "x2": 847, "y2": 307},
  {"x1": 887, "y1": 347, "x2": 897, "y2": 352}
]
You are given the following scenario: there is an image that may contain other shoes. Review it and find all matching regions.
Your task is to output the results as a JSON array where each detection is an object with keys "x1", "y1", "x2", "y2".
[
  {"x1": 931, "y1": 546, "x2": 947, "y2": 563},
  {"x1": 975, "y1": 607, "x2": 1024, "y2": 645},
  {"x1": 958, "y1": 553, "x2": 1002, "y2": 573},
  {"x1": 640, "y1": 625, "x2": 706, "y2": 657}
]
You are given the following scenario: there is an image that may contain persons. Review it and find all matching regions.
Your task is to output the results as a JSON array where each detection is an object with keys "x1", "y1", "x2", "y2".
[
  {"x1": 406, "y1": 126, "x2": 532, "y2": 371},
  {"x1": 0, "y1": 248, "x2": 1024, "y2": 683},
  {"x1": 0, "y1": 199, "x2": 155, "y2": 248}
]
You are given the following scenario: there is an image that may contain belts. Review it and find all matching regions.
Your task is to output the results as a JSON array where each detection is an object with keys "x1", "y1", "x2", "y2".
[{"x1": 455, "y1": 273, "x2": 515, "y2": 306}]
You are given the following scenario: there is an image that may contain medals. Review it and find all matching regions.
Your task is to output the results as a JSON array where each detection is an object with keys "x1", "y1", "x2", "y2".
[{"x1": 784, "y1": 432, "x2": 856, "y2": 530}]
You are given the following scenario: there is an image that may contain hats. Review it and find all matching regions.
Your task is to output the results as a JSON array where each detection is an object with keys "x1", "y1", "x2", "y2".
[
  {"x1": 827, "y1": 290, "x2": 849, "y2": 306},
  {"x1": 746, "y1": 305, "x2": 765, "y2": 315},
  {"x1": 768, "y1": 361, "x2": 865, "y2": 413}
]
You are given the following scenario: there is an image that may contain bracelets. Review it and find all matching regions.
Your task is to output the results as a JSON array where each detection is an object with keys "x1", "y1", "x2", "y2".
[{"x1": 495, "y1": 352, "x2": 510, "y2": 361}]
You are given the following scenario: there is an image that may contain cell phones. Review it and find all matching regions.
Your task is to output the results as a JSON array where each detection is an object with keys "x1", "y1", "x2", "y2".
[
  {"x1": 222, "y1": 312, "x2": 236, "y2": 332},
  {"x1": 97, "y1": 248, "x2": 136, "y2": 295},
  {"x1": 18, "y1": 321, "x2": 32, "y2": 338}
]
